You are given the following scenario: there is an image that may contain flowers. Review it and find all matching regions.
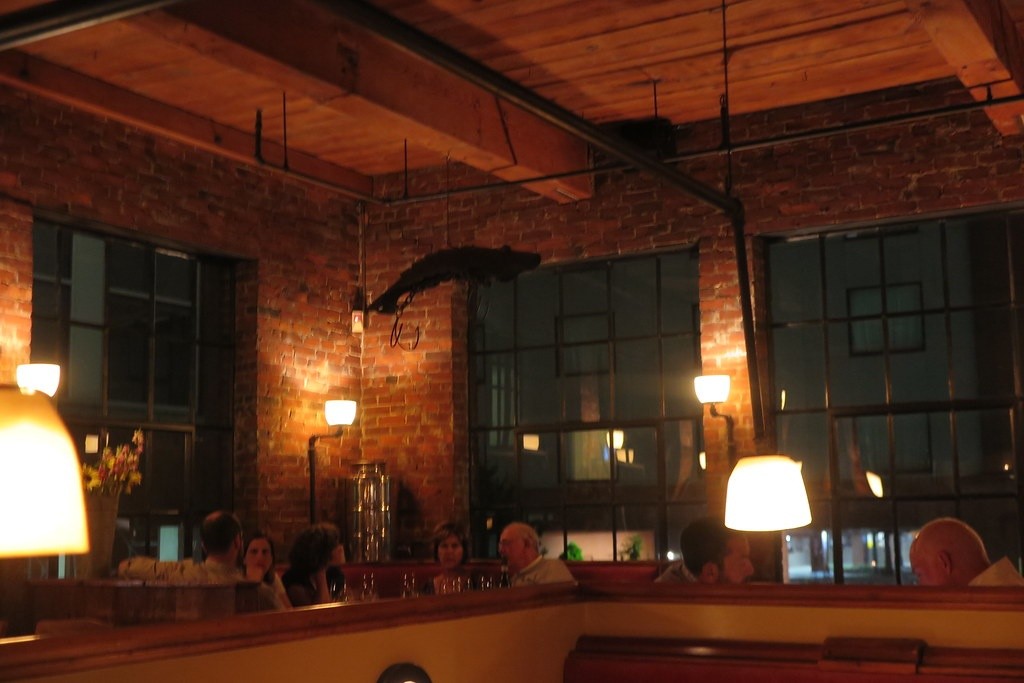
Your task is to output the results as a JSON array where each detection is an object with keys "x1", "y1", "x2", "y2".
[{"x1": 79, "y1": 429, "x2": 144, "y2": 497}]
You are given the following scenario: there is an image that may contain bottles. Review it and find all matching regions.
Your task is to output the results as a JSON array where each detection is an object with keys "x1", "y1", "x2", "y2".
[{"x1": 498, "y1": 556, "x2": 511, "y2": 590}]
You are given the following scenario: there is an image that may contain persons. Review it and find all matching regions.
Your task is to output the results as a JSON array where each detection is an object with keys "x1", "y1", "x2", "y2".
[
  {"x1": 910, "y1": 518, "x2": 1024, "y2": 586},
  {"x1": 498, "y1": 522, "x2": 572, "y2": 589},
  {"x1": 282, "y1": 523, "x2": 345, "y2": 607},
  {"x1": 119, "y1": 511, "x2": 292, "y2": 610},
  {"x1": 421, "y1": 523, "x2": 479, "y2": 596},
  {"x1": 654, "y1": 517, "x2": 754, "y2": 584}
]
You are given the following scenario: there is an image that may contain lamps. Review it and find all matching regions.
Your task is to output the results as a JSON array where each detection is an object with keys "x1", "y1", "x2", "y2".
[
  {"x1": 724, "y1": 456, "x2": 811, "y2": 582},
  {"x1": 695, "y1": 375, "x2": 734, "y2": 470},
  {"x1": 308, "y1": 400, "x2": 357, "y2": 524},
  {"x1": 0, "y1": 363, "x2": 90, "y2": 560}
]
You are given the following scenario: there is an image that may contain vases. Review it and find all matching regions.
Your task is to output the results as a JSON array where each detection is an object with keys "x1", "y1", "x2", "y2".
[{"x1": 73, "y1": 494, "x2": 119, "y2": 578}]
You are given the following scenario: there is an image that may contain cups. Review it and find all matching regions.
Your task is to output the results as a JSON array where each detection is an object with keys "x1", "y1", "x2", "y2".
[
  {"x1": 329, "y1": 578, "x2": 347, "y2": 603},
  {"x1": 363, "y1": 570, "x2": 374, "y2": 603},
  {"x1": 440, "y1": 576, "x2": 494, "y2": 594},
  {"x1": 401, "y1": 570, "x2": 414, "y2": 596}
]
